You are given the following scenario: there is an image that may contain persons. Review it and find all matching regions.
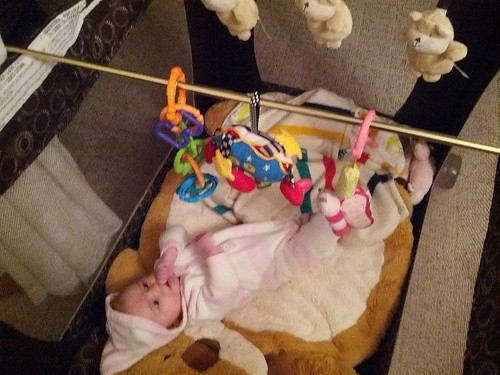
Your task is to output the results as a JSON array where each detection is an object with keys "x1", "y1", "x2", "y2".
[{"x1": 99, "y1": 180, "x2": 370, "y2": 375}]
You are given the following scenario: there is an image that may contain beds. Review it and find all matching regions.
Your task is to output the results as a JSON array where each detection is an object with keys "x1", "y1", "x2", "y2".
[{"x1": 0, "y1": 1, "x2": 500, "y2": 375}]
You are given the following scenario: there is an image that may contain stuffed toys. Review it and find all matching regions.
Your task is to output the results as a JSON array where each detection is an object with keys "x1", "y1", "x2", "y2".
[
  {"x1": 203, "y1": 125, "x2": 375, "y2": 236},
  {"x1": 203, "y1": 0, "x2": 468, "y2": 82},
  {"x1": 398, "y1": 137, "x2": 437, "y2": 204}
]
